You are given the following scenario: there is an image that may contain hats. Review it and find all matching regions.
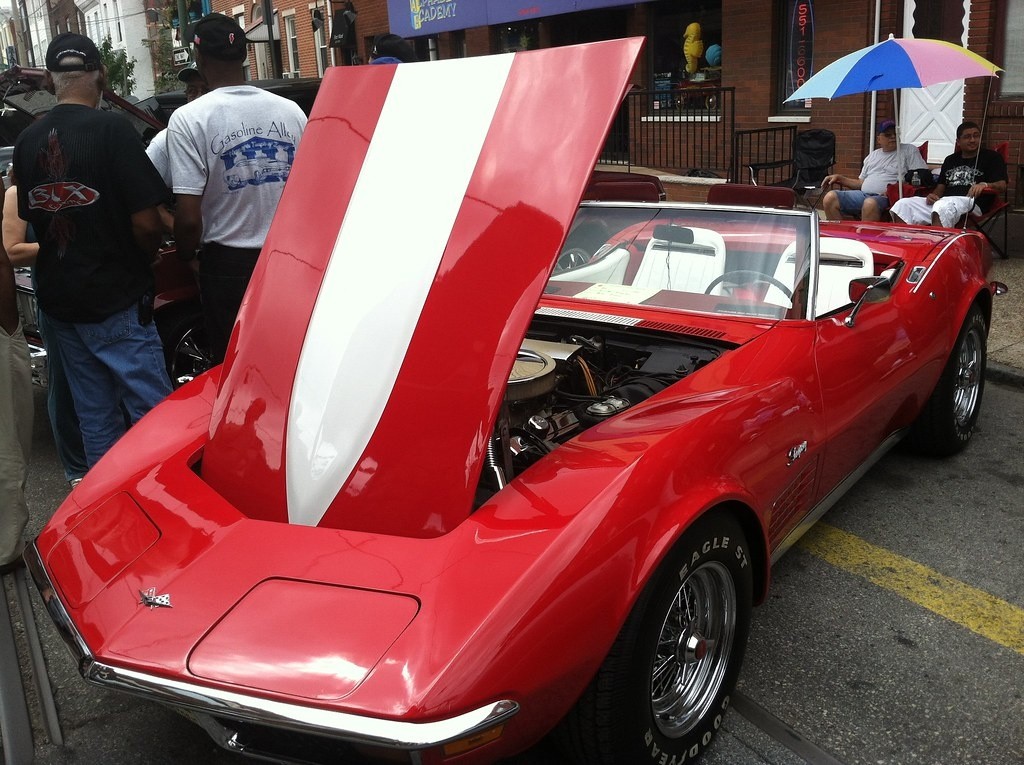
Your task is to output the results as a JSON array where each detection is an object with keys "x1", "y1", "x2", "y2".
[
  {"x1": 877, "y1": 120, "x2": 896, "y2": 134},
  {"x1": 45, "y1": 33, "x2": 99, "y2": 74},
  {"x1": 177, "y1": 61, "x2": 200, "y2": 83},
  {"x1": 369, "y1": 34, "x2": 416, "y2": 64},
  {"x1": 193, "y1": 12, "x2": 246, "y2": 59},
  {"x1": 371, "y1": 55, "x2": 401, "y2": 64}
]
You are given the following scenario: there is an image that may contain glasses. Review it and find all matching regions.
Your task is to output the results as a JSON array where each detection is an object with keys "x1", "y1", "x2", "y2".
[
  {"x1": 184, "y1": 86, "x2": 208, "y2": 97},
  {"x1": 877, "y1": 132, "x2": 895, "y2": 138},
  {"x1": 960, "y1": 134, "x2": 981, "y2": 141}
]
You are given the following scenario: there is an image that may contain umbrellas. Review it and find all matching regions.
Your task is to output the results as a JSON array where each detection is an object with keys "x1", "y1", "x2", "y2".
[{"x1": 783, "y1": 34, "x2": 1006, "y2": 198}]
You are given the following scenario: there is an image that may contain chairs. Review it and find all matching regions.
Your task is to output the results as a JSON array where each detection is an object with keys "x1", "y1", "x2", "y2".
[
  {"x1": 632, "y1": 226, "x2": 726, "y2": 295},
  {"x1": 742, "y1": 128, "x2": 837, "y2": 222},
  {"x1": 763, "y1": 238, "x2": 875, "y2": 315},
  {"x1": 829, "y1": 139, "x2": 1010, "y2": 259}
]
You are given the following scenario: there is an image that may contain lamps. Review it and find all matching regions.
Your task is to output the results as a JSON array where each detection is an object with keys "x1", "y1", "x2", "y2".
[
  {"x1": 343, "y1": 0, "x2": 358, "y2": 27},
  {"x1": 312, "y1": 0, "x2": 325, "y2": 33}
]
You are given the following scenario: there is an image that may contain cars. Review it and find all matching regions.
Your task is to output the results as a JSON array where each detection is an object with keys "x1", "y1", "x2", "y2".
[{"x1": 0, "y1": 61, "x2": 327, "y2": 406}]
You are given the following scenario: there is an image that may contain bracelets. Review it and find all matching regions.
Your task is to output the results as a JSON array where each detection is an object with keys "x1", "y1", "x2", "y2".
[{"x1": 980, "y1": 182, "x2": 988, "y2": 188}]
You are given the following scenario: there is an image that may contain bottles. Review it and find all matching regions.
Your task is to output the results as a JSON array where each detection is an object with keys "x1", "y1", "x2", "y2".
[{"x1": 911, "y1": 172, "x2": 920, "y2": 186}]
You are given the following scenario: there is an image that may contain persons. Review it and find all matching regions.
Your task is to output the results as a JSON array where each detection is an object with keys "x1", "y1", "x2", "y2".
[
  {"x1": 821, "y1": 121, "x2": 935, "y2": 222},
  {"x1": 145, "y1": 62, "x2": 209, "y2": 303},
  {"x1": 13, "y1": 33, "x2": 174, "y2": 469},
  {"x1": 167, "y1": 13, "x2": 309, "y2": 365},
  {"x1": 2, "y1": 111, "x2": 89, "y2": 488},
  {"x1": 368, "y1": 33, "x2": 418, "y2": 64},
  {"x1": 0, "y1": 177, "x2": 34, "y2": 576},
  {"x1": 889, "y1": 121, "x2": 1008, "y2": 228}
]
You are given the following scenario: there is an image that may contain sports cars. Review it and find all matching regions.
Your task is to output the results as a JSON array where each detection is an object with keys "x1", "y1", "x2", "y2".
[{"x1": 22, "y1": 34, "x2": 1010, "y2": 764}]
